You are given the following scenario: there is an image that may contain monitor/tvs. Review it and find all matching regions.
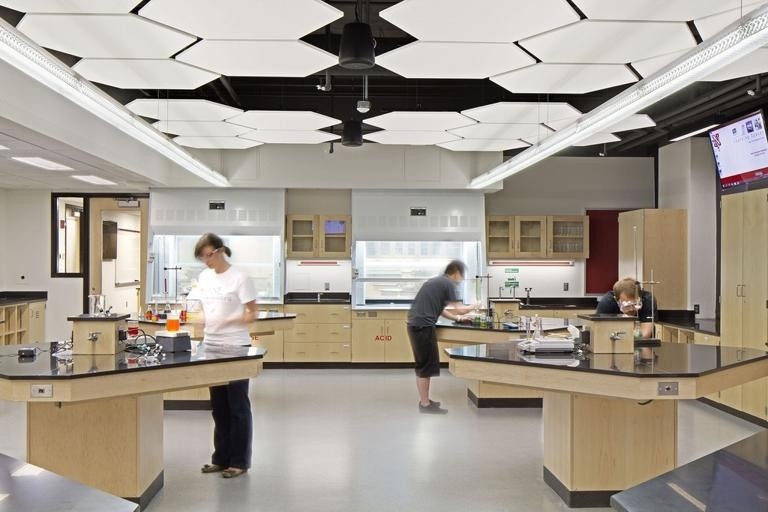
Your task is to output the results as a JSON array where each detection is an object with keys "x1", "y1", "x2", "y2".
[
  {"x1": 705, "y1": 108, "x2": 768, "y2": 197},
  {"x1": 103, "y1": 220, "x2": 117, "y2": 258}
]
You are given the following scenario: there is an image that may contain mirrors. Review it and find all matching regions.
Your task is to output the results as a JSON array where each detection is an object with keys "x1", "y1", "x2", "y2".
[{"x1": 50, "y1": 191, "x2": 83, "y2": 277}]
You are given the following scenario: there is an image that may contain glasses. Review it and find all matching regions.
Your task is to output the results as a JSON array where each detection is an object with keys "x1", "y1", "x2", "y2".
[{"x1": 198, "y1": 248, "x2": 220, "y2": 262}]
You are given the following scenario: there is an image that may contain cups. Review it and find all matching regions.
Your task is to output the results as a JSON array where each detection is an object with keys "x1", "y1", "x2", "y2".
[
  {"x1": 127, "y1": 320, "x2": 139, "y2": 338},
  {"x1": 166, "y1": 314, "x2": 180, "y2": 333},
  {"x1": 127, "y1": 355, "x2": 138, "y2": 368}
]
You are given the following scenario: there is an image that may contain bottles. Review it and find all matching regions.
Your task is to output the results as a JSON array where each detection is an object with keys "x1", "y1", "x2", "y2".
[
  {"x1": 553, "y1": 223, "x2": 583, "y2": 253},
  {"x1": 519, "y1": 314, "x2": 545, "y2": 342},
  {"x1": 474, "y1": 309, "x2": 488, "y2": 329},
  {"x1": 137, "y1": 304, "x2": 171, "y2": 321},
  {"x1": 635, "y1": 348, "x2": 642, "y2": 366},
  {"x1": 180, "y1": 304, "x2": 187, "y2": 322},
  {"x1": 635, "y1": 321, "x2": 642, "y2": 339}
]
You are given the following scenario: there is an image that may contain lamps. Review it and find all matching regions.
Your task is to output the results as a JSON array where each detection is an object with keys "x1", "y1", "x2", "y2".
[
  {"x1": 466, "y1": 1, "x2": 768, "y2": 193},
  {"x1": 336, "y1": 1, "x2": 377, "y2": 71},
  {"x1": 356, "y1": 73, "x2": 372, "y2": 115},
  {"x1": 0, "y1": 14, "x2": 233, "y2": 189},
  {"x1": 340, "y1": 79, "x2": 365, "y2": 147}
]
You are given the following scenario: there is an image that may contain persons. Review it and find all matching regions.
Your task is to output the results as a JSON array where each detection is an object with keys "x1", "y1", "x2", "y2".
[
  {"x1": 183, "y1": 232, "x2": 257, "y2": 478},
  {"x1": 714, "y1": 134, "x2": 721, "y2": 147},
  {"x1": 595, "y1": 277, "x2": 657, "y2": 340},
  {"x1": 407, "y1": 257, "x2": 480, "y2": 414}
]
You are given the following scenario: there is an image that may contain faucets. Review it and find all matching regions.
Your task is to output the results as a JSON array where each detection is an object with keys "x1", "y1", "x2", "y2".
[{"x1": 498, "y1": 285, "x2": 504, "y2": 298}]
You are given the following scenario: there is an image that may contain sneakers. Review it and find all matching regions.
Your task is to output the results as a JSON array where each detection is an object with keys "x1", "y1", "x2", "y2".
[
  {"x1": 222, "y1": 468, "x2": 247, "y2": 477},
  {"x1": 203, "y1": 464, "x2": 227, "y2": 473},
  {"x1": 419, "y1": 400, "x2": 448, "y2": 414}
]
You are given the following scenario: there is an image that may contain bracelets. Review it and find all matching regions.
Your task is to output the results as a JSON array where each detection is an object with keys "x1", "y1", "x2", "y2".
[{"x1": 456, "y1": 315, "x2": 462, "y2": 322}]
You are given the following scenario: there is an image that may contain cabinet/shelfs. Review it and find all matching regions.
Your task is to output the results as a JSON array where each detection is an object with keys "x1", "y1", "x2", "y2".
[
  {"x1": 29, "y1": 299, "x2": 46, "y2": 342},
  {"x1": 618, "y1": 208, "x2": 688, "y2": 309},
  {"x1": 486, "y1": 214, "x2": 546, "y2": 259},
  {"x1": 547, "y1": 213, "x2": 589, "y2": 260},
  {"x1": 250, "y1": 305, "x2": 284, "y2": 361},
  {"x1": 0, "y1": 301, "x2": 28, "y2": 347},
  {"x1": 663, "y1": 324, "x2": 720, "y2": 404},
  {"x1": 285, "y1": 304, "x2": 352, "y2": 362},
  {"x1": 352, "y1": 310, "x2": 415, "y2": 363},
  {"x1": 286, "y1": 214, "x2": 351, "y2": 260},
  {"x1": 720, "y1": 189, "x2": 768, "y2": 428}
]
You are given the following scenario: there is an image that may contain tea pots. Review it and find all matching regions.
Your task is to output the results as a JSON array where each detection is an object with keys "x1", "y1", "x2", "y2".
[{"x1": 88, "y1": 294, "x2": 106, "y2": 315}]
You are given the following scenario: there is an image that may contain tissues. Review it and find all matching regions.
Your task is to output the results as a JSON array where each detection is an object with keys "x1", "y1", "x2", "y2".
[{"x1": 567, "y1": 324, "x2": 583, "y2": 345}]
